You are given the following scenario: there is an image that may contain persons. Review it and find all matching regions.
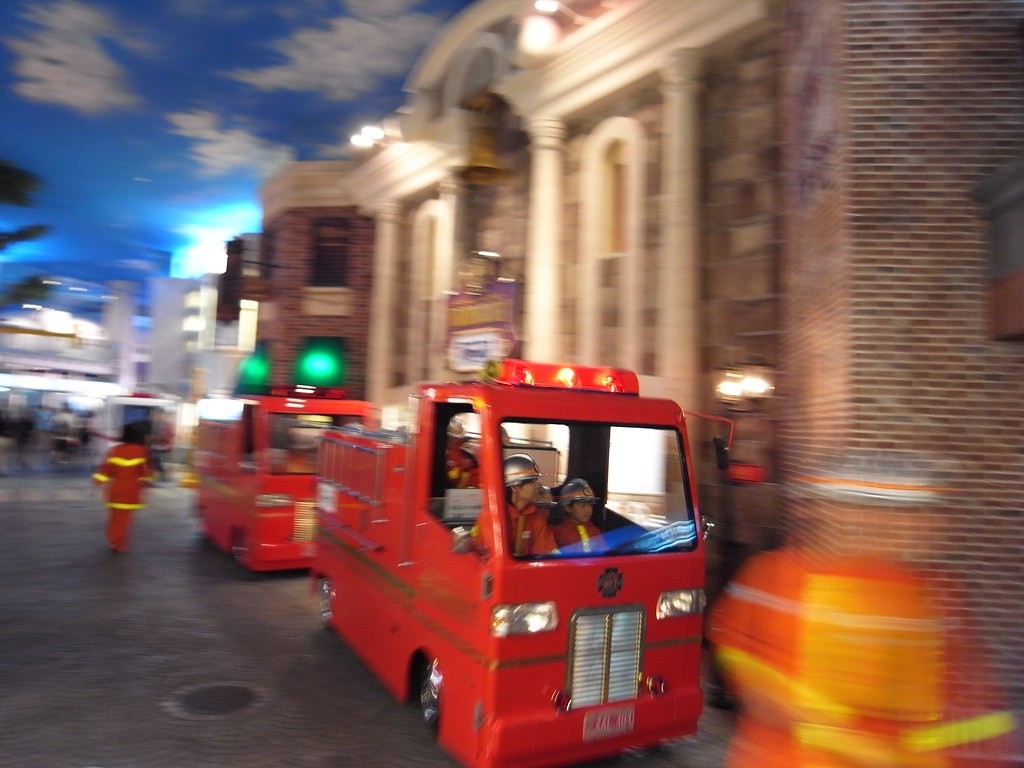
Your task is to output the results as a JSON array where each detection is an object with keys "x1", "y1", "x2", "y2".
[
  {"x1": 29, "y1": 403, "x2": 72, "y2": 429},
  {"x1": 91, "y1": 422, "x2": 155, "y2": 553},
  {"x1": 707, "y1": 455, "x2": 1018, "y2": 768},
  {"x1": 445, "y1": 416, "x2": 510, "y2": 487},
  {"x1": 469, "y1": 452, "x2": 557, "y2": 558},
  {"x1": 550, "y1": 478, "x2": 604, "y2": 556}
]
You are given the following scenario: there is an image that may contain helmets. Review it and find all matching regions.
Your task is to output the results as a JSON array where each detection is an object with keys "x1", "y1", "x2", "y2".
[
  {"x1": 446, "y1": 419, "x2": 465, "y2": 438},
  {"x1": 557, "y1": 478, "x2": 596, "y2": 507},
  {"x1": 502, "y1": 452, "x2": 547, "y2": 487},
  {"x1": 500, "y1": 425, "x2": 510, "y2": 448},
  {"x1": 460, "y1": 438, "x2": 480, "y2": 460}
]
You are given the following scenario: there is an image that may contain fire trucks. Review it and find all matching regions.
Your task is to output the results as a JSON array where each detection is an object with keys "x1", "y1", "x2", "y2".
[
  {"x1": 316, "y1": 356, "x2": 717, "y2": 768},
  {"x1": 187, "y1": 385, "x2": 382, "y2": 578}
]
